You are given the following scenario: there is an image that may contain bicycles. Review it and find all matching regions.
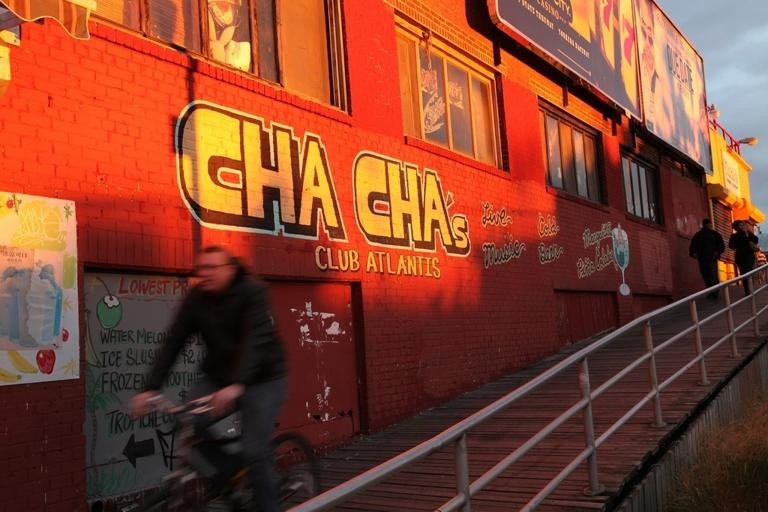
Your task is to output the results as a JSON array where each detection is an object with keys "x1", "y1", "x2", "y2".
[{"x1": 146, "y1": 394, "x2": 321, "y2": 512}]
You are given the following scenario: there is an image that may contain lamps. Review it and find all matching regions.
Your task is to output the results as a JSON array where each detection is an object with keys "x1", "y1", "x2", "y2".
[
  {"x1": 707, "y1": 104, "x2": 720, "y2": 118},
  {"x1": 729, "y1": 137, "x2": 758, "y2": 147}
]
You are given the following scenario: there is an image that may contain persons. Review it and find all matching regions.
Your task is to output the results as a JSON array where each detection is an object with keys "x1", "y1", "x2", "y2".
[
  {"x1": 689, "y1": 218, "x2": 726, "y2": 301},
  {"x1": 127, "y1": 244, "x2": 295, "y2": 512},
  {"x1": 638, "y1": 0, "x2": 713, "y2": 174},
  {"x1": 729, "y1": 219, "x2": 759, "y2": 296},
  {"x1": 755, "y1": 249, "x2": 768, "y2": 284}
]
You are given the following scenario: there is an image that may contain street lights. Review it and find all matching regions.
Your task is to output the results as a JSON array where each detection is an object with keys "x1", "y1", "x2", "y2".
[{"x1": 730, "y1": 137, "x2": 758, "y2": 147}]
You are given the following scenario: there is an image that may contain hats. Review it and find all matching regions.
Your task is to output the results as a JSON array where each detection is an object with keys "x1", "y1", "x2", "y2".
[{"x1": 732, "y1": 221, "x2": 744, "y2": 227}]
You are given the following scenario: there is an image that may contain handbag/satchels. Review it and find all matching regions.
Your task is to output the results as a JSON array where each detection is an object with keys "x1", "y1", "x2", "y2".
[{"x1": 745, "y1": 231, "x2": 759, "y2": 253}]
